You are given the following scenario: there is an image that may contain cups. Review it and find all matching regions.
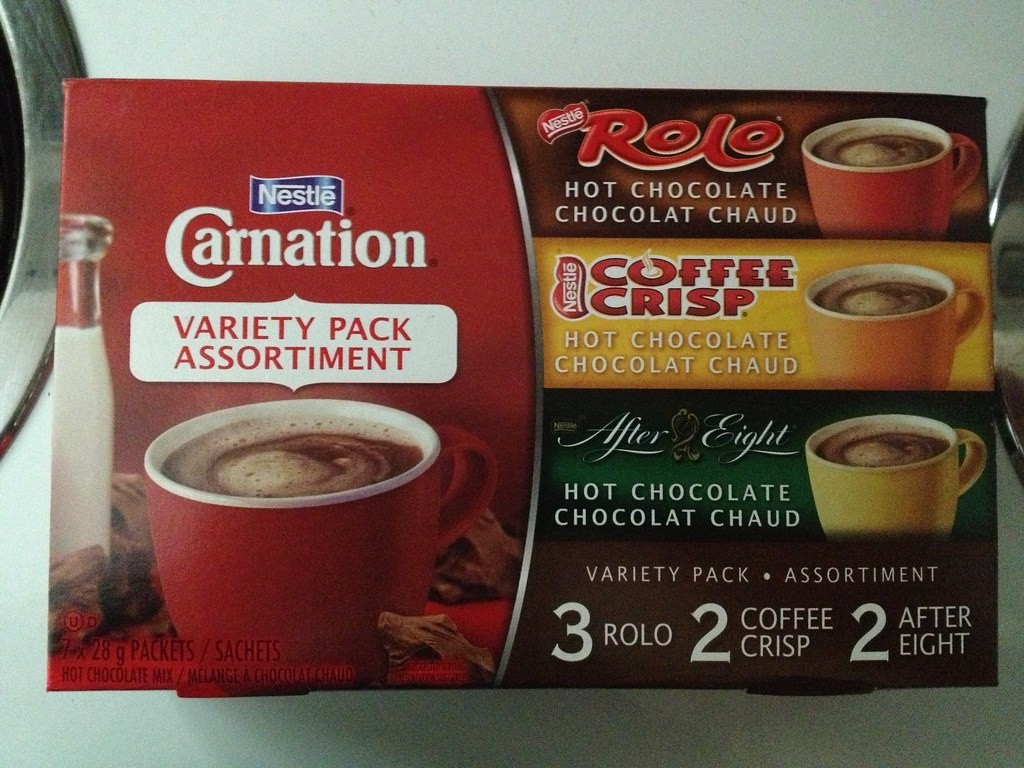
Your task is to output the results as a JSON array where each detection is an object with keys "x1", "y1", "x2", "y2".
[
  {"x1": 803, "y1": 263, "x2": 985, "y2": 390},
  {"x1": 804, "y1": 414, "x2": 987, "y2": 537},
  {"x1": 142, "y1": 397, "x2": 496, "y2": 679},
  {"x1": 800, "y1": 116, "x2": 982, "y2": 240}
]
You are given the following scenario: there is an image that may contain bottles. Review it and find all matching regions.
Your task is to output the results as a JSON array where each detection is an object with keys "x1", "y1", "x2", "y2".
[{"x1": 50, "y1": 214, "x2": 118, "y2": 643}]
